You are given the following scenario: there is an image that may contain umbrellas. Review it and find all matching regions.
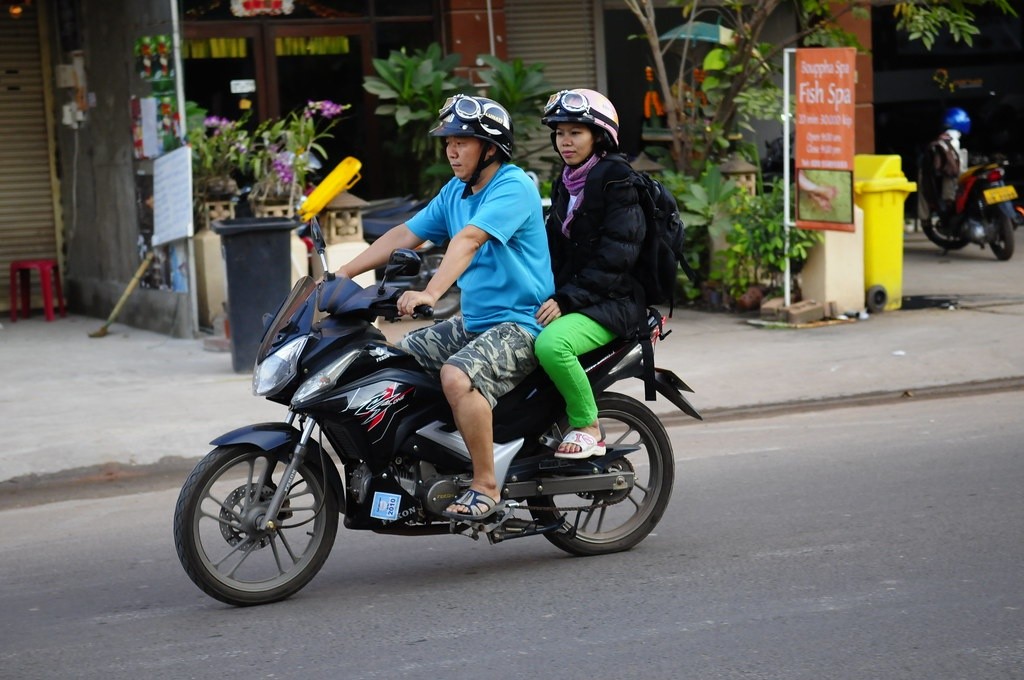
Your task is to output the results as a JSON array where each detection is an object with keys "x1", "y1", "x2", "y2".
[{"x1": 658, "y1": 16, "x2": 773, "y2": 77}]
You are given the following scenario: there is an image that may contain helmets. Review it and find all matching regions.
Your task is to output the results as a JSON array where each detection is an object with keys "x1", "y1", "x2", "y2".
[
  {"x1": 428, "y1": 96, "x2": 514, "y2": 161},
  {"x1": 542, "y1": 88, "x2": 619, "y2": 147},
  {"x1": 941, "y1": 107, "x2": 972, "y2": 135}
]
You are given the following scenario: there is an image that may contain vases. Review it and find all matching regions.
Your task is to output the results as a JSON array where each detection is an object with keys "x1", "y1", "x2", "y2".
[
  {"x1": 259, "y1": 195, "x2": 291, "y2": 219},
  {"x1": 207, "y1": 177, "x2": 236, "y2": 202}
]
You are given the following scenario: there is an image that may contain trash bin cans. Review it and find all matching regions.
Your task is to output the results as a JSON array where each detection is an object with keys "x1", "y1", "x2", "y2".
[
  {"x1": 212, "y1": 217, "x2": 300, "y2": 374},
  {"x1": 854, "y1": 154, "x2": 917, "y2": 311}
]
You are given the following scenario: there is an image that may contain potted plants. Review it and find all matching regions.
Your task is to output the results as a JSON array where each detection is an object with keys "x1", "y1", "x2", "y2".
[{"x1": 666, "y1": 163, "x2": 826, "y2": 309}]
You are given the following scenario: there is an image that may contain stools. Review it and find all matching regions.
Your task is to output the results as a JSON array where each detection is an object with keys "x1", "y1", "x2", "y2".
[{"x1": 6, "y1": 258, "x2": 65, "y2": 322}]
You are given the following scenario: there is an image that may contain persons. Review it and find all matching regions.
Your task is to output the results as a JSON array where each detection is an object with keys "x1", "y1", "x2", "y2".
[
  {"x1": 534, "y1": 88, "x2": 648, "y2": 459},
  {"x1": 315, "y1": 92, "x2": 557, "y2": 520}
]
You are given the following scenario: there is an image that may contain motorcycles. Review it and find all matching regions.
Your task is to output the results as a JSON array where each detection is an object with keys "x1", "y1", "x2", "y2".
[
  {"x1": 921, "y1": 109, "x2": 1024, "y2": 261},
  {"x1": 173, "y1": 215, "x2": 705, "y2": 606}
]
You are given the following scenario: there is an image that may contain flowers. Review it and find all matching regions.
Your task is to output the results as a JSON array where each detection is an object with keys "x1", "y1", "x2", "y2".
[{"x1": 190, "y1": 93, "x2": 354, "y2": 202}]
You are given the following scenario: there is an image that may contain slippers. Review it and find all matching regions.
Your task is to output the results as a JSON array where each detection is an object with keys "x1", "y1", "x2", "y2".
[
  {"x1": 442, "y1": 489, "x2": 505, "y2": 520},
  {"x1": 554, "y1": 431, "x2": 607, "y2": 459}
]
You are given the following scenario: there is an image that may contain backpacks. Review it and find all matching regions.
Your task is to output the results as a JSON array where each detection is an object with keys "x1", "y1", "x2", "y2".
[{"x1": 585, "y1": 154, "x2": 685, "y2": 304}]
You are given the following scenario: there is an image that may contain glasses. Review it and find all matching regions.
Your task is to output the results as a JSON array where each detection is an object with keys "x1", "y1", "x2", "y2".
[
  {"x1": 544, "y1": 89, "x2": 591, "y2": 115},
  {"x1": 438, "y1": 93, "x2": 486, "y2": 124}
]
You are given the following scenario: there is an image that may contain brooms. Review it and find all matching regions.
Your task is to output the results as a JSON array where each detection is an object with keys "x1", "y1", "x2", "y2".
[{"x1": 88, "y1": 252, "x2": 154, "y2": 338}]
